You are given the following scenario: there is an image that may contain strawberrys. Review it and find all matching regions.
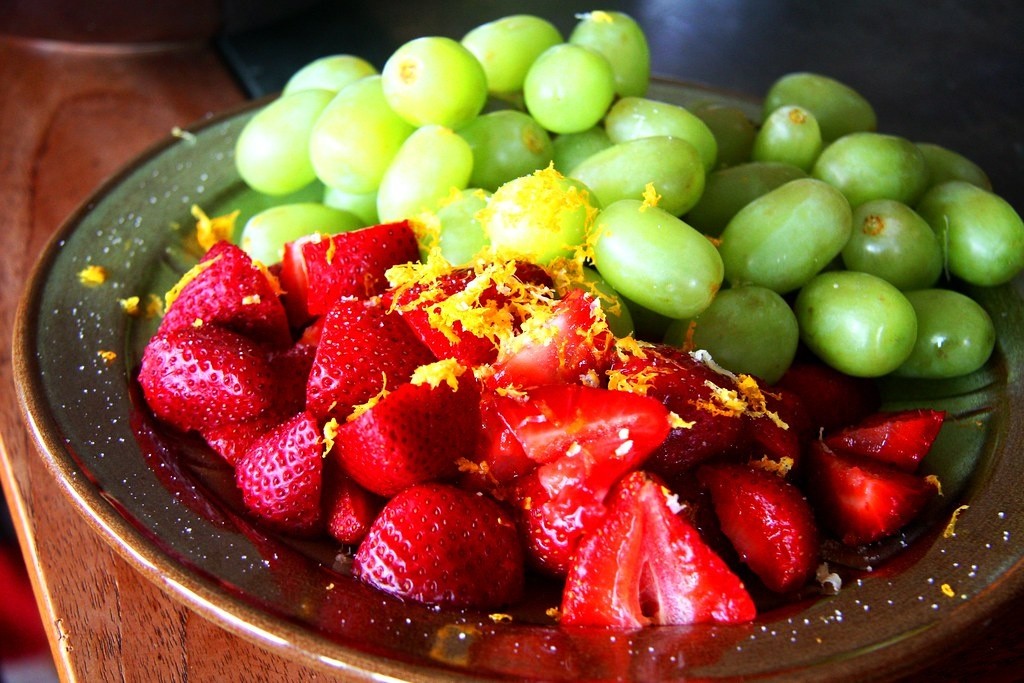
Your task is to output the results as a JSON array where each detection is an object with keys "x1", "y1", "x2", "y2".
[{"x1": 138, "y1": 216, "x2": 945, "y2": 630}]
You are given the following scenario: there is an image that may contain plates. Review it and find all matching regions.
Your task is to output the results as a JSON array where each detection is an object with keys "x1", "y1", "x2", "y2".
[{"x1": 8, "y1": 71, "x2": 1024, "y2": 683}]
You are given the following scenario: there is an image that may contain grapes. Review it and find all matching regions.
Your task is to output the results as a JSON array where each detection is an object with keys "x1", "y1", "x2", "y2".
[{"x1": 231, "y1": 9, "x2": 1024, "y2": 384}]
